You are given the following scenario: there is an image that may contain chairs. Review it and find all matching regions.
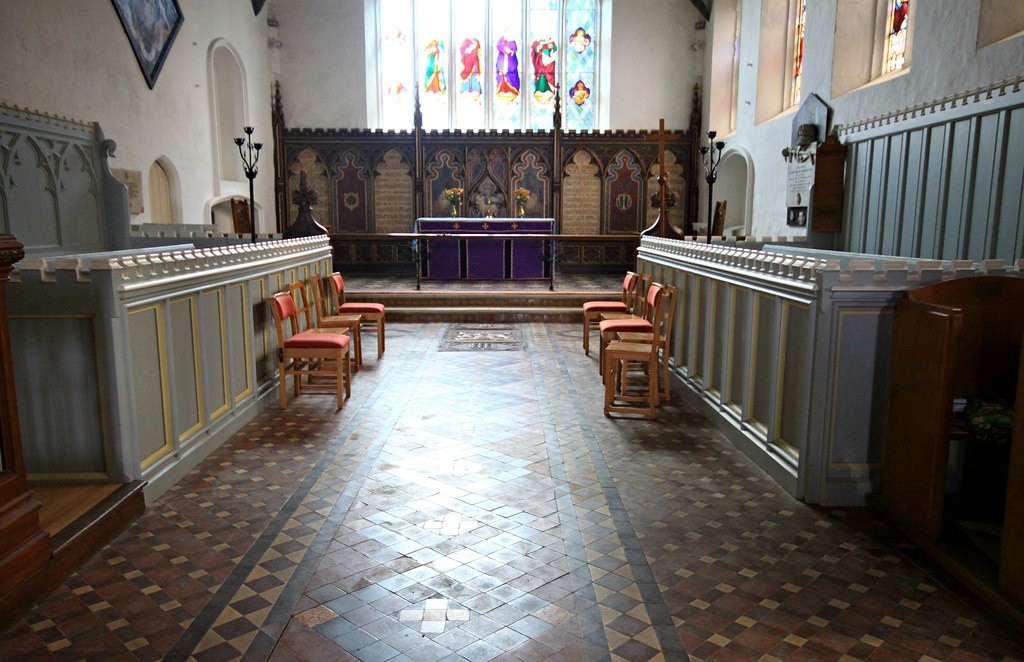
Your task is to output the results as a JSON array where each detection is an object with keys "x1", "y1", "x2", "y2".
[
  {"x1": 263, "y1": 289, "x2": 352, "y2": 411},
  {"x1": 599, "y1": 282, "x2": 663, "y2": 384},
  {"x1": 288, "y1": 281, "x2": 348, "y2": 391},
  {"x1": 308, "y1": 273, "x2": 362, "y2": 371},
  {"x1": 617, "y1": 282, "x2": 677, "y2": 397},
  {"x1": 326, "y1": 270, "x2": 387, "y2": 358},
  {"x1": 604, "y1": 295, "x2": 666, "y2": 418},
  {"x1": 582, "y1": 271, "x2": 637, "y2": 355},
  {"x1": 601, "y1": 272, "x2": 654, "y2": 323}
]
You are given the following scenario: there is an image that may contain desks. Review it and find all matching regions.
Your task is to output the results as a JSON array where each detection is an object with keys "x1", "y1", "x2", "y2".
[{"x1": 411, "y1": 217, "x2": 557, "y2": 281}]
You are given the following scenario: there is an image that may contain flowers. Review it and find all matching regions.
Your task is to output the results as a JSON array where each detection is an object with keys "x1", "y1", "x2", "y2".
[
  {"x1": 442, "y1": 185, "x2": 463, "y2": 206},
  {"x1": 511, "y1": 185, "x2": 530, "y2": 207}
]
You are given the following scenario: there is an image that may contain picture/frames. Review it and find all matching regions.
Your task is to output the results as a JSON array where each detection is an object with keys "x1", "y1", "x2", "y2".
[{"x1": 109, "y1": 0, "x2": 185, "y2": 90}]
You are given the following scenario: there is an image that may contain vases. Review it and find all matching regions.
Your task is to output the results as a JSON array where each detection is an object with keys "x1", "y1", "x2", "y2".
[
  {"x1": 518, "y1": 205, "x2": 525, "y2": 217},
  {"x1": 449, "y1": 205, "x2": 457, "y2": 216}
]
